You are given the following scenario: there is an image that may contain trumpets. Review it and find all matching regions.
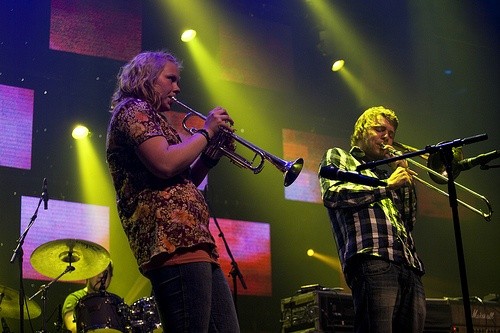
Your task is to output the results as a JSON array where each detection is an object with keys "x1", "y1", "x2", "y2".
[{"x1": 171, "y1": 96, "x2": 304, "y2": 187}]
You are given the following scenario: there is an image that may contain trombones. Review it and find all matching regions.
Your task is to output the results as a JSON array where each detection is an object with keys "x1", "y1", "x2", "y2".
[{"x1": 381, "y1": 140, "x2": 491, "y2": 220}]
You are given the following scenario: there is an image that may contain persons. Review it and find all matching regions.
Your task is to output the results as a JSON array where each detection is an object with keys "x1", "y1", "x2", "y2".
[
  {"x1": 318, "y1": 105, "x2": 427, "y2": 333},
  {"x1": 104, "y1": 49, "x2": 240, "y2": 333},
  {"x1": 61, "y1": 258, "x2": 127, "y2": 333}
]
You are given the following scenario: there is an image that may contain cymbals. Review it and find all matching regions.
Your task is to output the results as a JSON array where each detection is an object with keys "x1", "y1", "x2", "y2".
[
  {"x1": 31, "y1": 239, "x2": 111, "y2": 281},
  {"x1": 0, "y1": 282, "x2": 42, "y2": 319}
]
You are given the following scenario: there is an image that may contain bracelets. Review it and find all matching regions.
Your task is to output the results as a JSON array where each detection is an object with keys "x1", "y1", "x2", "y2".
[{"x1": 196, "y1": 128, "x2": 211, "y2": 142}]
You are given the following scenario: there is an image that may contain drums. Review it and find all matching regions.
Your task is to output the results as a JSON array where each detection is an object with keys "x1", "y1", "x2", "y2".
[
  {"x1": 74, "y1": 292, "x2": 130, "y2": 333},
  {"x1": 131, "y1": 295, "x2": 163, "y2": 333}
]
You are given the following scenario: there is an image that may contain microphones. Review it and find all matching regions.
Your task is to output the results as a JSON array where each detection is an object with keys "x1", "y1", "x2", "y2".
[
  {"x1": 43, "y1": 180, "x2": 48, "y2": 209},
  {"x1": 459, "y1": 150, "x2": 500, "y2": 171},
  {"x1": 99, "y1": 270, "x2": 108, "y2": 292},
  {"x1": 204, "y1": 185, "x2": 207, "y2": 201}
]
